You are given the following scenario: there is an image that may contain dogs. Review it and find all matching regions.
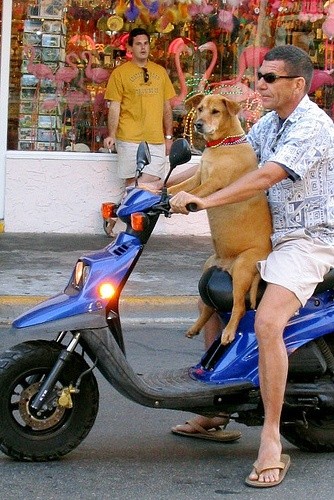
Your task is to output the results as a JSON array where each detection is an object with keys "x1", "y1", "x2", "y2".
[{"x1": 123, "y1": 91, "x2": 274, "y2": 345}]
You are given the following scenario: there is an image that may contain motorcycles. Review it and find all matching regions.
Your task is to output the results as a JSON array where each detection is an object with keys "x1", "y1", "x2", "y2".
[{"x1": 0, "y1": 138, "x2": 334, "y2": 462}]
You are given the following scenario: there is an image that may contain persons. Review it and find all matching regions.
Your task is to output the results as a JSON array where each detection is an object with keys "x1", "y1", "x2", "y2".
[
  {"x1": 103, "y1": 27, "x2": 176, "y2": 156},
  {"x1": 138, "y1": 45, "x2": 334, "y2": 489}
]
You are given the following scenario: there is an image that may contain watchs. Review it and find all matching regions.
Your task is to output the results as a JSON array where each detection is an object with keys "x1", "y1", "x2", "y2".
[{"x1": 164, "y1": 135, "x2": 173, "y2": 140}]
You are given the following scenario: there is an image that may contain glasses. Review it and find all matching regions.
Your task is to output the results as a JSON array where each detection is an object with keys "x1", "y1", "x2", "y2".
[
  {"x1": 257, "y1": 72, "x2": 300, "y2": 83},
  {"x1": 141, "y1": 68, "x2": 148, "y2": 83}
]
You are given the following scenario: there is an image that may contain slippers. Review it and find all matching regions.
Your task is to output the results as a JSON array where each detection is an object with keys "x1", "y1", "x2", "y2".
[
  {"x1": 244, "y1": 454, "x2": 291, "y2": 488},
  {"x1": 170, "y1": 420, "x2": 241, "y2": 442},
  {"x1": 102, "y1": 218, "x2": 116, "y2": 238}
]
